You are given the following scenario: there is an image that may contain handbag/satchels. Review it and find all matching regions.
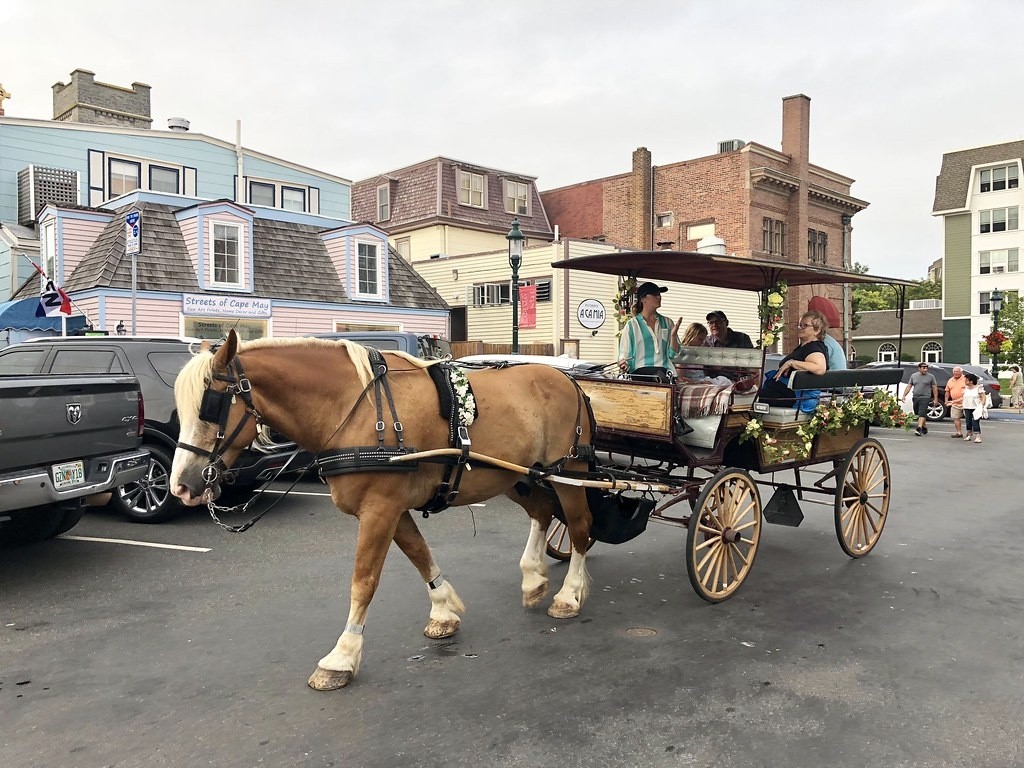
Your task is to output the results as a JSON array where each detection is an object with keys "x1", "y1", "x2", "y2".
[{"x1": 759, "y1": 379, "x2": 796, "y2": 408}]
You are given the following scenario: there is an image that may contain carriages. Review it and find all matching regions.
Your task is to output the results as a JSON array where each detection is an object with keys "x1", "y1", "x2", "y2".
[{"x1": 169, "y1": 250, "x2": 926, "y2": 693}]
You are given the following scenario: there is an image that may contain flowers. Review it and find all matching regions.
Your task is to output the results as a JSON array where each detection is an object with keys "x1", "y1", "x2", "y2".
[
  {"x1": 612, "y1": 277, "x2": 639, "y2": 339},
  {"x1": 737, "y1": 382, "x2": 919, "y2": 465},
  {"x1": 756, "y1": 278, "x2": 790, "y2": 351}
]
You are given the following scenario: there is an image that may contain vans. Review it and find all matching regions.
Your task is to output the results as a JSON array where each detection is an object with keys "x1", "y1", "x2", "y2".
[{"x1": 303, "y1": 330, "x2": 454, "y2": 361}]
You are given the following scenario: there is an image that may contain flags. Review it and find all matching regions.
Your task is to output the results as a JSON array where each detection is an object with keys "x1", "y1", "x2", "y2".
[{"x1": 34, "y1": 263, "x2": 71, "y2": 318}]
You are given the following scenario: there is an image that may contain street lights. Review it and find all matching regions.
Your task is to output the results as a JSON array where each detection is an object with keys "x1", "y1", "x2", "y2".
[
  {"x1": 989, "y1": 287, "x2": 1004, "y2": 381},
  {"x1": 503, "y1": 218, "x2": 525, "y2": 355}
]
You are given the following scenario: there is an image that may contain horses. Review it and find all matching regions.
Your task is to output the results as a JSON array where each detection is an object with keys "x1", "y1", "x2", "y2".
[{"x1": 167, "y1": 329, "x2": 594, "y2": 692}]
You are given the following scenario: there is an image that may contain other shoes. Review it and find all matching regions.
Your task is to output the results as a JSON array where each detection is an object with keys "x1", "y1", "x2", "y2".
[
  {"x1": 974, "y1": 437, "x2": 982, "y2": 443},
  {"x1": 915, "y1": 427, "x2": 922, "y2": 436},
  {"x1": 951, "y1": 433, "x2": 963, "y2": 438},
  {"x1": 964, "y1": 435, "x2": 971, "y2": 440},
  {"x1": 921, "y1": 426, "x2": 928, "y2": 434}
]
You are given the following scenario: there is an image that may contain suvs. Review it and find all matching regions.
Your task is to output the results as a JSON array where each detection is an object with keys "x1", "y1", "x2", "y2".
[{"x1": 0, "y1": 334, "x2": 316, "y2": 526}]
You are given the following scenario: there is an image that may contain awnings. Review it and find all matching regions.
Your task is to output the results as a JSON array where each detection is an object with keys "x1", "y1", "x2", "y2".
[{"x1": 0, "y1": 297, "x2": 86, "y2": 332}]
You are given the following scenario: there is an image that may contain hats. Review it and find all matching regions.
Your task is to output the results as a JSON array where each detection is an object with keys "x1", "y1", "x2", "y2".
[
  {"x1": 705, "y1": 311, "x2": 726, "y2": 321},
  {"x1": 636, "y1": 282, "x2": 668, "y2": 300}
]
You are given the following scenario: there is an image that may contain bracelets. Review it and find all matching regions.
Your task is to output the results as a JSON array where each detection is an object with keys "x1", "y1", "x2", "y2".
[{"x1": 790, "y1": 359, "x2": 793, "y2": 367}]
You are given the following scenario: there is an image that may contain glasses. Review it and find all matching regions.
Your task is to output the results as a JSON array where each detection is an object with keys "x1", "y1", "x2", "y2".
[{"x1": 798, "y1": 323, "x2": 814, "y2": 329}]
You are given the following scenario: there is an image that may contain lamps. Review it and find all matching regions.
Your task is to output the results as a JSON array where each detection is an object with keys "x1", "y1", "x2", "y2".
[{"x1": 452, "y1": 269, "x2": 458, "y2": 280}]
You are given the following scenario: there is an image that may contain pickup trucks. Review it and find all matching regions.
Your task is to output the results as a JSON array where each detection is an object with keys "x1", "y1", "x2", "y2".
[{"x1": 0, "y1": 370, "x2": 155, "y2": 543}]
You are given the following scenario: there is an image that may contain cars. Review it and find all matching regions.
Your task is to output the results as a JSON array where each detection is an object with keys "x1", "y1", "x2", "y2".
[
  {"x1": 451, "y1": 352, "x2": 611, "y2": 379},
  {"x1": 845, "y1": 359, "x2": 1003, "y2": 423}
]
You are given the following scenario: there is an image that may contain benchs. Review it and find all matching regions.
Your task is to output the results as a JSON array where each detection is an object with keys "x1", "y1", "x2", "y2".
[
  {"x1": 671, "y1": 382, "x2": 735, "y2": 461},
  {"x1": 755, "y1": 367, "x2": 905, "y2": 465},
  {"x1": 672, "y1": 345, "x2": 767, "y2": 411}
]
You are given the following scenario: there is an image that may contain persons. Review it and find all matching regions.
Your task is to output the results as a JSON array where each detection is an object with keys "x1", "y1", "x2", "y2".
[
  {"x1": 902, "y1": 362, "x2": 938, "y2": 436},
  {"x1": 677, "y1": 323, "x2": 707, "y2": 380},
  {"x1": 1009, "y1": 367, "x2": 1024, "y2": 408},
  {"x1": 945, "y1": 367, "x2": 973, "y2": 438},
  {"x1": 946, "y1": 373, "x2": 986, "y2": 442},
  {"x1": 701, "y1": 310, "x2": 758, "y2": 386},
  {"x1": 808, "y1": 296, "x2": 847, "y2": 401},
  {"x1": 764, "y1": 312, "x2": 828, "y2": 413},
  {"x1": 619, "y1": 282, "x2": 694, "y2": 435}
]
